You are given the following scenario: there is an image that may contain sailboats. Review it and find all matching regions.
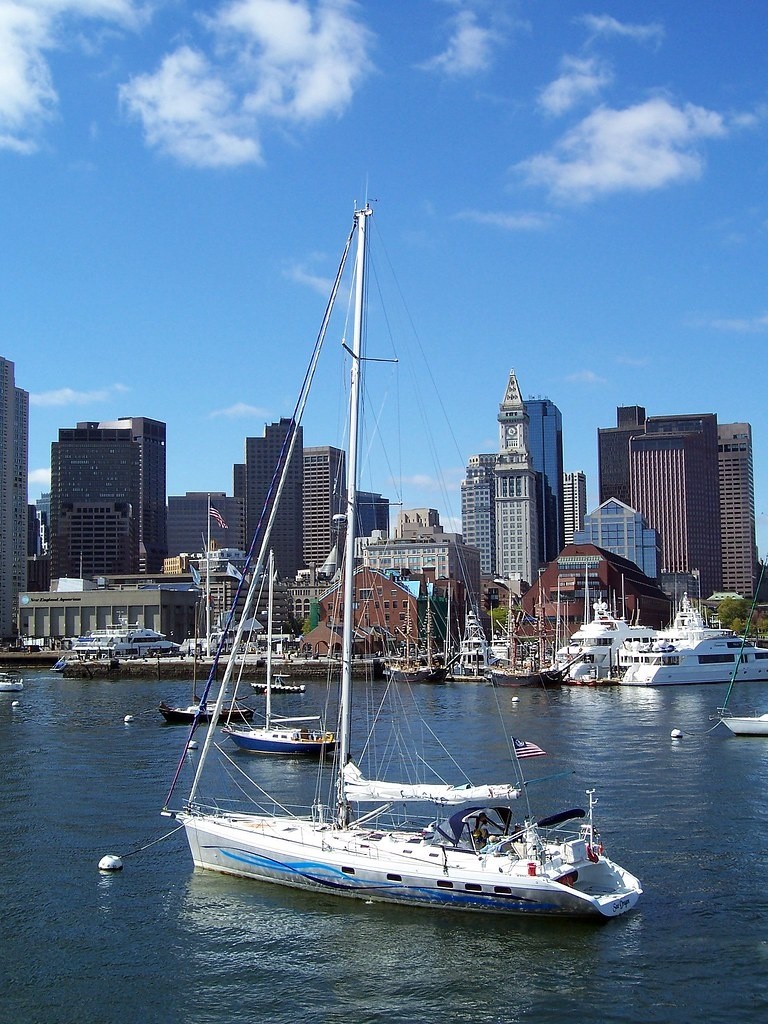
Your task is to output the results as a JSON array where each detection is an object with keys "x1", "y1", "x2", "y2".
[
  {"x1": 718, "y1": 552, "x2": 768, "y2": 737},
  {"x1": 157, "y1": 599, "x2": 255, "y2": 720},
  {"x1": 159, "y1": 202, "x2": 644, "y2": 922},
  {"x1": 224, "y1": 549, "x2": 341, "y2": 753},
  {"x1": 381, "y1": 572, "x2": 570, "y2": 688}
]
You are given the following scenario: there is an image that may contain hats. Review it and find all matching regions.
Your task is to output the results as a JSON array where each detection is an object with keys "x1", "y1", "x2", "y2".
[{"x1": 514, "y1": 823, "x2": 521, "y2": 827}]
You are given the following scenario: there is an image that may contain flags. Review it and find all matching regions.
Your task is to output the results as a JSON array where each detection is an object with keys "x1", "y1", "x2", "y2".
[
  {"x1": 209, "y1": 507, "x2": 229, "y2": 529},
  {"x1": 227, "y1": 562, "x2": 242, "y2": 580},
  {"x1": 190, "y1": 565, "x2": 202, "y2": 585},
  {"x1": 511, "y1": 736, "x2": 548, "y2": 760}
]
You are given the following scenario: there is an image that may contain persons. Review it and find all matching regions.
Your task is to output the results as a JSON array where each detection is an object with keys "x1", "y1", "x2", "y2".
[
  {"x1": 511, "y1": 823, "x2": 523, "y2": 843},
  {"x1": 475, "y1": 813, "x2": 489, "y2": 849}
]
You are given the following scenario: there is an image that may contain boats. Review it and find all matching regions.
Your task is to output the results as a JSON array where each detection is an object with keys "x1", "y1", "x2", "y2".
[
  {"x1": 556, "y1": 570, "x2": 768, "y2": 685},
  {"x1": 0, "y1": 669, "x2": 23, "y2": 692},
  {"x1": 48, "y1": 617, "x2": 181, "y2": 678},
  {"x1": 250, "y1": 674, "x2": 308, "y2": 694}
]
